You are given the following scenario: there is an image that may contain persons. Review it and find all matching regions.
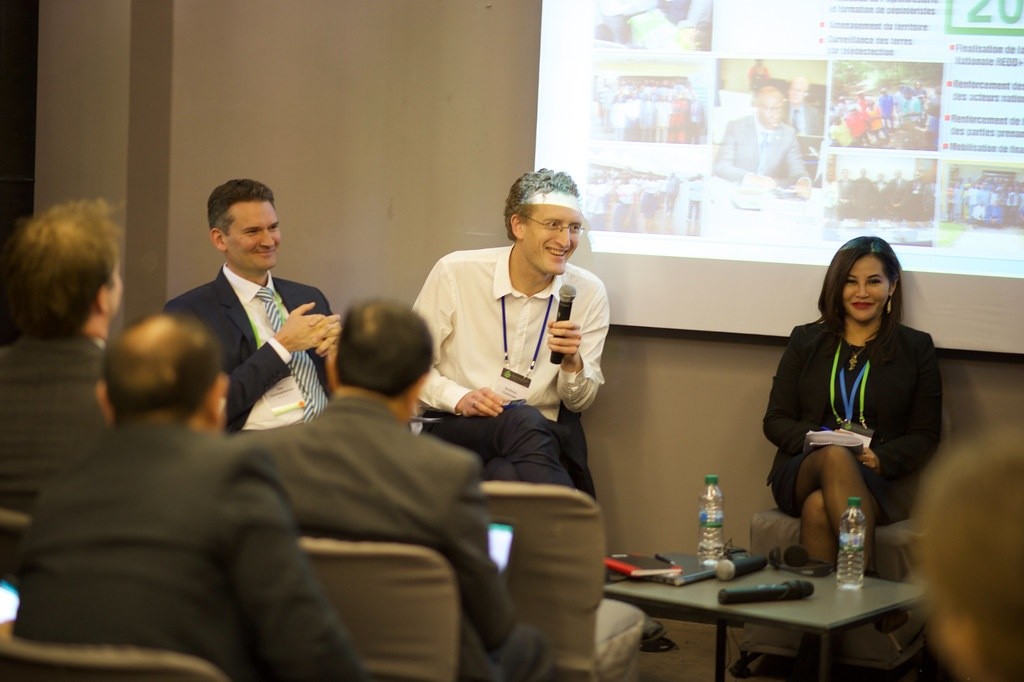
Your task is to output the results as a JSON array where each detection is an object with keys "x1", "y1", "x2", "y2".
[
  {"x1": 909, "y1": 396, "x2": 1024, "y2": 682},
  {"x1": 411, "y1": 167, "x2": 675, "y2": 654},
  {"x1": 0, "y1": 198, "x2": 123, "y2": 575},
  {"x1": 573, "y1": 0, "x2": 1024, "y2": 228},
  {"x1": 165, "y1": 178, "x2": 341, "y2": 433},
  {"x1": 0, "y1": 309, "x2": 376, "y2": 682},
  {"x1": 763, "y1": 235, "x2": 944, "y2": 682},
  {"x1": 246, "y1": 297, "x2": 562, "y2": 682}
]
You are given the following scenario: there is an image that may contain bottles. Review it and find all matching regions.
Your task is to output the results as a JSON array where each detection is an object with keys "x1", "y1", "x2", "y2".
[
  {"x1": 836, "y1": 496, "x2": 866, "y2": 590},
  {"x1": 697, "y1": 475, "x2": 725, "y2": 570}
]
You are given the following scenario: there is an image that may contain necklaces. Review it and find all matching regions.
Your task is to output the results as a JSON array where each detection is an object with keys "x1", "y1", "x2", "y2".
[{"x1": 847, "y1": 344, "x2": 866, "y2": 370}]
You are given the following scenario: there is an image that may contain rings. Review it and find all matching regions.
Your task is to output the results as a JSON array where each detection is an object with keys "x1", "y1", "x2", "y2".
[{"x1": 472, "y1": 400, "x2": 477, "y2": 408}]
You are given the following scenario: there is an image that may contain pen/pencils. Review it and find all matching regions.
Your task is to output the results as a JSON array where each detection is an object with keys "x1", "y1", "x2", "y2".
[{"x1": 655, "y1": 553, "x2": 676, "y2": 566}]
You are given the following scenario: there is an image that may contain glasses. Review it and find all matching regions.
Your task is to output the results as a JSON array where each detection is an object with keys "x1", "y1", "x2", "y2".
[{"x1": 521, "y1": 215, "x2": 585, "y2": 237}]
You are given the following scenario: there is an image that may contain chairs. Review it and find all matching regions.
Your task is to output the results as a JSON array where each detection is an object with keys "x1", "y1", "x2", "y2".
[
  {"x1": 729, "y1": 473, "x2": 928, "y2": 682},
  {"x1": 0, "y1": 483, "x2": 647, "y2": 682}
]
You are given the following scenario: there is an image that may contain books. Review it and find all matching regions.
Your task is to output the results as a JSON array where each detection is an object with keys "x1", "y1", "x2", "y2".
[{"x1": 600, "y1": 553, "x2": 716, "y2": 586}]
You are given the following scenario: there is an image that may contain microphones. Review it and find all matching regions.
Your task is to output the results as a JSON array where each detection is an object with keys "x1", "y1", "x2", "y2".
[
  {"x1": 718, "y1": 580, "x2": 814, "y2": 604},
  {"x1": 717, "y1": 556, "x2": 769, "y2": 582},
  {"x1": 550, "y1": 285, "x2": 578, "y2": 365},
  {"x1": 783, "y1": 545, "x2": 825, "y2": 567}
]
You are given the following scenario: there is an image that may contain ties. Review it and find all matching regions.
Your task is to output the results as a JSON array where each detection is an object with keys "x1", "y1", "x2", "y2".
[
  {"x1": 254, "y1": 286, "x2": 328, "y2": 424},
  {"x1": 758, "y1": 132, "x2": 769, "y2": 175},
  {"x1": 791, "y1": 110, "x2": 800, "y2": 134}
]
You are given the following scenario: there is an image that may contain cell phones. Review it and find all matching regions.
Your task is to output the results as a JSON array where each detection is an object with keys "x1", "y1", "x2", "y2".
[
  {"x1": 486, "y1": 520, "x2": 514, "y2": 570},
  {"x1": 502, "y1": 399, "x2": 526, "y2": 410}
]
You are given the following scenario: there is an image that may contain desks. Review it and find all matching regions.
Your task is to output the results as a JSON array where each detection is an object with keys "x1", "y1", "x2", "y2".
[{"x1": 606, "y1": 553, "x2": 927, "y2": 682}]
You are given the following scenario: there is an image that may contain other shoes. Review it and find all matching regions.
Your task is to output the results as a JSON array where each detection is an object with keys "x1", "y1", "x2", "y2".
[
  {"x1": 642, "y1": 617, "x2": 664, "y2": 638},
  {"x1": 863, "y1": 567, "x2": 910, "y2": 635}
]
